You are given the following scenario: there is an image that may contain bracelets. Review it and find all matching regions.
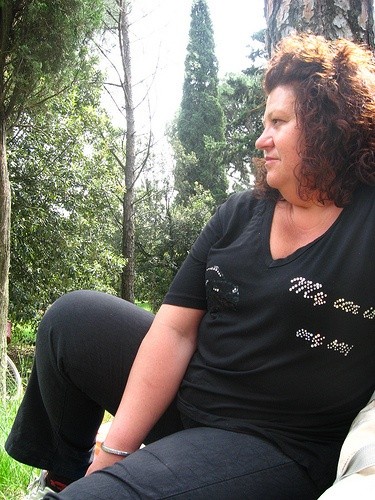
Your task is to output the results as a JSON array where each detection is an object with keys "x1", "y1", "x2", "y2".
[{"x1": 99, "y1": 442, "x2": 132, "y2": 458}]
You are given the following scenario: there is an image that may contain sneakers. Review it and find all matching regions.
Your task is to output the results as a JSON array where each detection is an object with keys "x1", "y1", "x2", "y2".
[{"x1": 22, "y1": 470, "x2": 55, "y2": 500}]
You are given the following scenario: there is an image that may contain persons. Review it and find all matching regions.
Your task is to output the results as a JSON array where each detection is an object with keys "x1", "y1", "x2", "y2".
[{"x1": 4, "y1": 30, "x2": 375, "y2": 500}]
